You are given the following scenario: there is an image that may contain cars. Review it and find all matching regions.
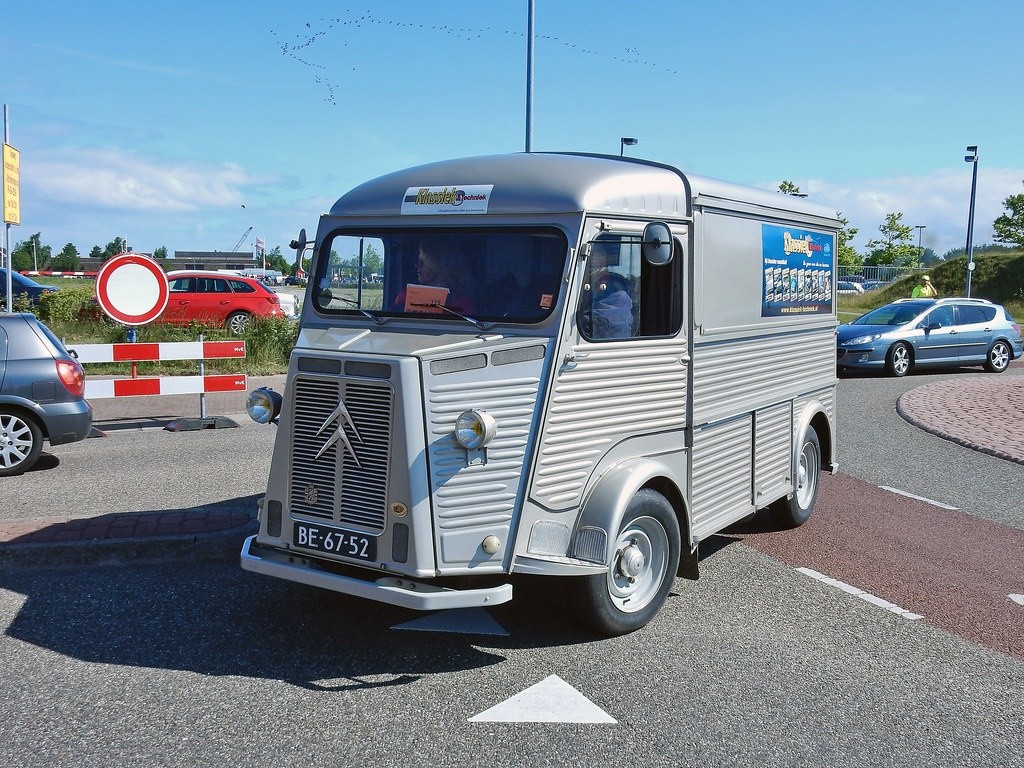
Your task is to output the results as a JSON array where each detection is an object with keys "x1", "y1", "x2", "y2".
[
  {"x1": 838, "y1": 275, "x2": 893, "y2": 295},
  {"x1": 0, "y1": 312, "x2": 93, "y2": 477},
  {"x1": 78, "y1": 269, "x2": 282, "y2": 336},
  {"x1": 0, "y1": 267, "x2": 60, "y2": 308},
  {"x1": 838, "y1": 298, "x2": 1024, "y2": 376},
  {"x1": 243, "y1": 274, "x2": 359, "y2": 286}
]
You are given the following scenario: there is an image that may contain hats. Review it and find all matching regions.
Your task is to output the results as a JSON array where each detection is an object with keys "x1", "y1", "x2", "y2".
[{"x1": 921, "y1": 274, "x2": 930, "y2": 281}]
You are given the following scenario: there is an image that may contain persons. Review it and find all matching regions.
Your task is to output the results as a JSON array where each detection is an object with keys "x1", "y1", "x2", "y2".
[
  {"x1": 578, "y1": 245, "x2": 633, "y2": 338},
  {"x1": 394, "y1": 237, "x2": 473, "y2": 314},
  {"x1": 912, "y1": 274, "x2": 937, "y2": 298}
]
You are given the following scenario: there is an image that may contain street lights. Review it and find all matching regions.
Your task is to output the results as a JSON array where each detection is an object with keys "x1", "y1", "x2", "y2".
[
  {"x1": 915, "y1": 225, "x2": 926, "y2": 269},
  {"x1": 963, "y1": 144, "x2": 979, "y2": 302}
]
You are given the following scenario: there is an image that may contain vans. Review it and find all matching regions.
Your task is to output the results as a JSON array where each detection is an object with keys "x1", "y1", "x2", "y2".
[{"x1": 240, "y1": 149, "x2": 849, "y2": 638}]
